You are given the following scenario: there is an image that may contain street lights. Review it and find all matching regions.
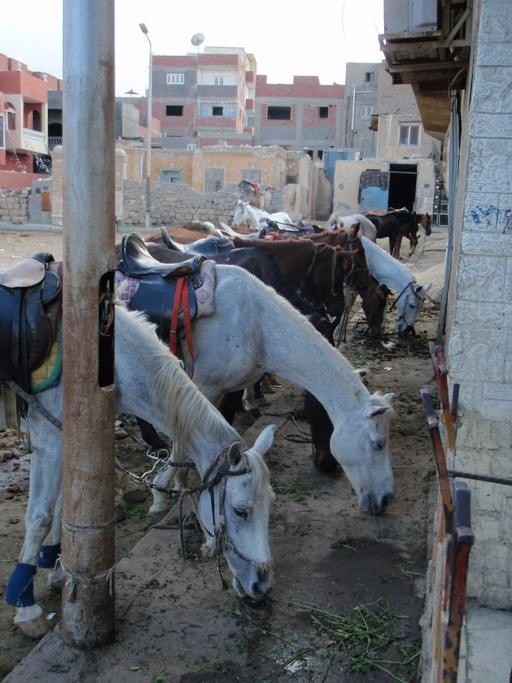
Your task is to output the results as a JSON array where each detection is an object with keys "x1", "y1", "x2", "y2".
[{"x1": 139, "y1": 23, "x2": 152, "y2": 228}]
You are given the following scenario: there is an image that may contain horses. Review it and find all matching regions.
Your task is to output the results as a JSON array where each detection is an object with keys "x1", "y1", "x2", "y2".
[{"x1": 0, "y1": 197, "x2": 434, "y2": 639}]
